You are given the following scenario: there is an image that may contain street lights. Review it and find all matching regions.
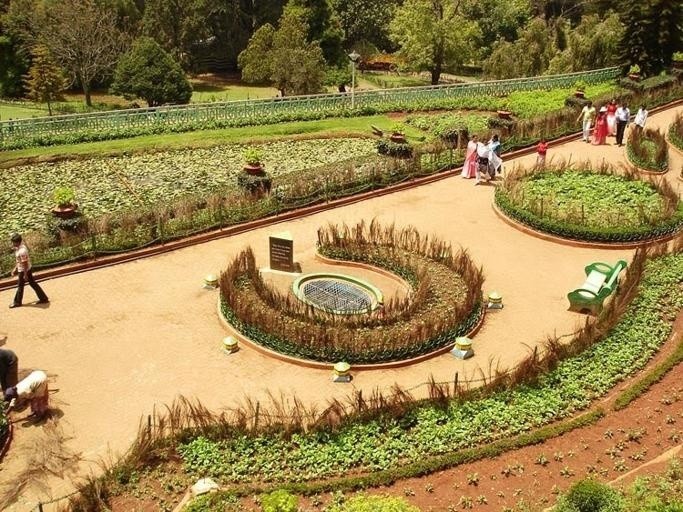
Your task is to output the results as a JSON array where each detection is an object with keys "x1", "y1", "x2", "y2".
[{"x1": 348, "y1": 50, "x2": 360, "y2": 110}]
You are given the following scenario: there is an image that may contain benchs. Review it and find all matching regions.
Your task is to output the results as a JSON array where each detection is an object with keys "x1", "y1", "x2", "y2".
[{"x1": 566, "y1": 259, "x2": 626, "y2": 318}]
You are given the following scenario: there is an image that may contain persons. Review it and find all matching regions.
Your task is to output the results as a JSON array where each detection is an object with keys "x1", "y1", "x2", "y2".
[
  {"x1": 8, "y1": 233, "x2": 48, "y2": 309},
  {"x1": 461, "y1": 134, "x2": 478, "y2": 180},
  {"x1": 2, "y1": 370, "x2": 50, "y2": 426},
  {"x1": 487, "y1": 134, "x2": 502, "y2": 180},
  {"x1": 535, "y1": 137, "x2": 548, "y2": 166},
  {"x1": 576, "y1": 98, "x2": 648, "y2": 148},
  {"x1": 473, "y1": 141, "x2": 493, "y2": 184},
  {"x1": 0, "y1": 335, "x2": 19, "y2": 395}
]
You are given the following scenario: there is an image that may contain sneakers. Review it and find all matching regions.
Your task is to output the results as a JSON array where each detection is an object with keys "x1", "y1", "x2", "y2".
[
  {"x1": 9, "y1": 302, "x2": 21, "y2": 308},
  {"x1": 36, "y1": 299, "x2": 49, "y2": 304}
]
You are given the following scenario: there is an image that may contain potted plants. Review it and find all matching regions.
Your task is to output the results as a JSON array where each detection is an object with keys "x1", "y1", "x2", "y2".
[
  {"x1": 498, "y1": 98, "x2": 511, "y2": 119},
  {"x1": 627, "y1": 64, "x2": 639, "y2": 80},
  {"x1": 672, "y1": 51, "x2": 682, "y2": 67},
  {"x1": 574, "y1": 80, "x2": 585, "y2": 100},
  {"x1": 51, "y1": 184, "x2": 76, "y2": 215},
  {"x1": 392, "y1": 122, "x2": 405, "y2": 142},
  {"x1": 242, "y1": 148, "x2": 263, "y2": 176}
]
